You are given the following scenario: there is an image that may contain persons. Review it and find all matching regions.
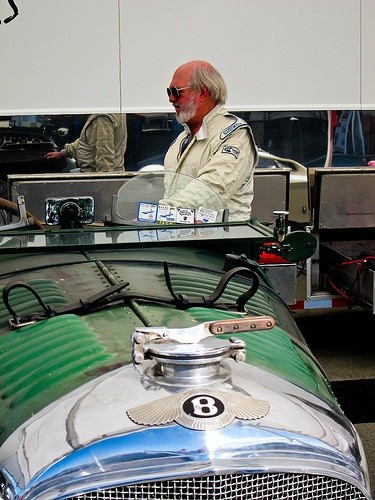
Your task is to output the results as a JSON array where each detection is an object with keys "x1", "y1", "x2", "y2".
[
  {"x1": 134, "y1": 61, "x2": 260, "y2": 222},
  {"x1": 45, "y1": 113, "x2": 127, "y2": 172}
]
